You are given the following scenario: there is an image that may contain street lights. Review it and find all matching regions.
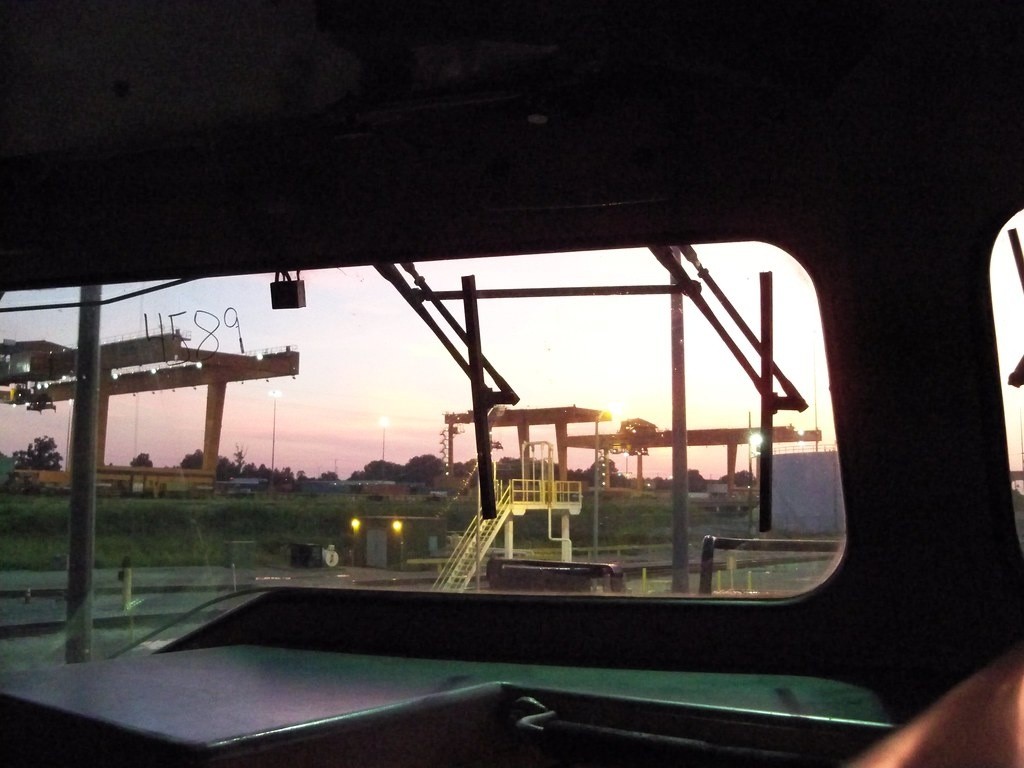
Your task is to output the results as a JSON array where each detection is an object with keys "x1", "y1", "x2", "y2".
[
  {"x1": 746, "y1": 430, "x2": 763, "y2": 537},
  {"x1": 377, "y1": 416, "x2": 387, "y2": 495},
  {"x1": 269, "y1": 390, "x2": 280, "y2": 495}
]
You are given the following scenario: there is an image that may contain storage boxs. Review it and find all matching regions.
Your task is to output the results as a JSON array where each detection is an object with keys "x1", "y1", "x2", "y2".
[{"x1": 289, "y1": 543, "x2": 323, "y2": 568}]
